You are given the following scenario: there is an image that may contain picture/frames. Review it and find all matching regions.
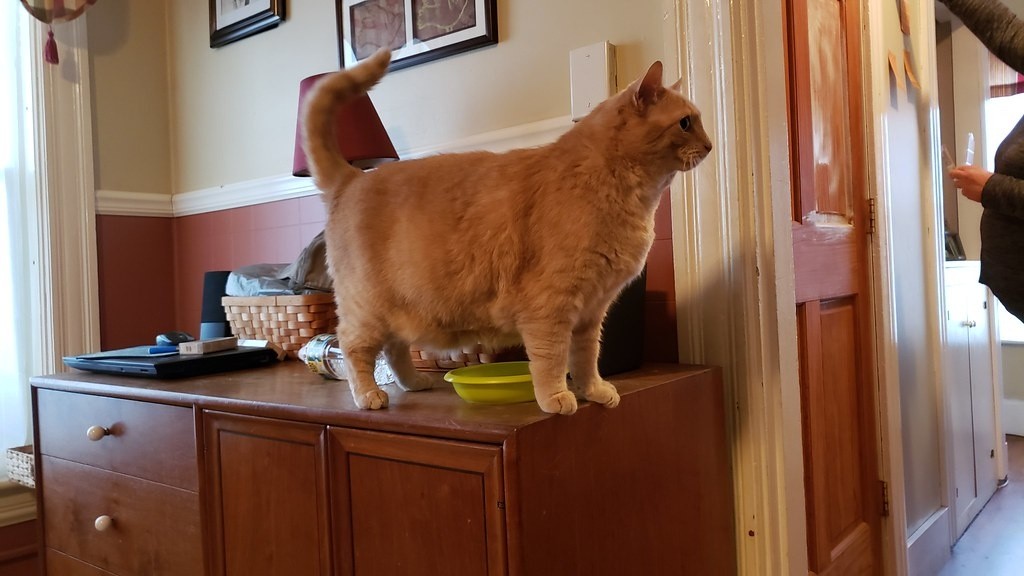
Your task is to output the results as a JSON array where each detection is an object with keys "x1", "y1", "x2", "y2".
[
  {"x1": 334, "y1": 0, "x2": 499, "y2": 74},
  {"x1": 208, "y1": 0, "x2": 284, "y2": 49}
]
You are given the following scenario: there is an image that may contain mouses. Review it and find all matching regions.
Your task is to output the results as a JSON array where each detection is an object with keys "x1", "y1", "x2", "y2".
[{"x1": 156, "y1": 331, "x2": 196, "y2": 347}]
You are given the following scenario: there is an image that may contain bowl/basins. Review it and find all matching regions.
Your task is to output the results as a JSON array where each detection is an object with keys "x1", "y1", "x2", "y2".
[{"x1": 443, "y1": 361, "x2": 537, "y2": 402}]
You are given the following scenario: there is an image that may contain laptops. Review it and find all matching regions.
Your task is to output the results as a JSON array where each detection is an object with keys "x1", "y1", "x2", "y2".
[{"x1": 63, "y1": 346, "x2": 279, "y2": 377}]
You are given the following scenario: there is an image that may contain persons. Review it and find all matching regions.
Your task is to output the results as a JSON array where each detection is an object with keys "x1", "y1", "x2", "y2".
[{"x1": 937, "y1": 0, "x2": 1023, "y2": 324}]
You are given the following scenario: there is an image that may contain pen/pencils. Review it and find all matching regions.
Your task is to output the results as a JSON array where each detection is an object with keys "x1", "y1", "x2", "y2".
[{"x1": 149, "y1": 346, "x2": 180, "y2": 353}]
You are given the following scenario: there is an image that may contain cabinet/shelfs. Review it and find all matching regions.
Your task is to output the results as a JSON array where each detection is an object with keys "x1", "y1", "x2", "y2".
[
  {"x1": 943, "y1": 259, "x2": 1000, "y2": 549},
  {"x1": 26, "y1": 358, "x2": 739, "y2": 576}
]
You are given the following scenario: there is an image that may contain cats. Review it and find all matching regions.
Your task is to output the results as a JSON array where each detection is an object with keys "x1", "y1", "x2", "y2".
[{"x1": 298, "y1": 46, "x2": 712, "y2": 414}]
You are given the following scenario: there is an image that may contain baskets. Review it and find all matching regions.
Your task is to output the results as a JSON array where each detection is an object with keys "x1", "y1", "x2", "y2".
[
  {"x1": 221, "y1": 296, "x2": 335, "y2": 359},
  {"x1": 6, "y1": 445, "x2": 36, "y2": 488},
  {"x1": 405, "y1": 339, "x2": 497, "y2": 372}
]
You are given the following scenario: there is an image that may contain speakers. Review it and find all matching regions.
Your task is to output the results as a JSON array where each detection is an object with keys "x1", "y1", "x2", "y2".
[{"x1": 199, "y1": 270, "x2": 234, "y2": 341}]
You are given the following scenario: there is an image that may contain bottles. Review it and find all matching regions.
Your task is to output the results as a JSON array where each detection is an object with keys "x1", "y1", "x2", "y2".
[{"x1": 297, "y1": 333, "x2": 395, "y2": 385}]
